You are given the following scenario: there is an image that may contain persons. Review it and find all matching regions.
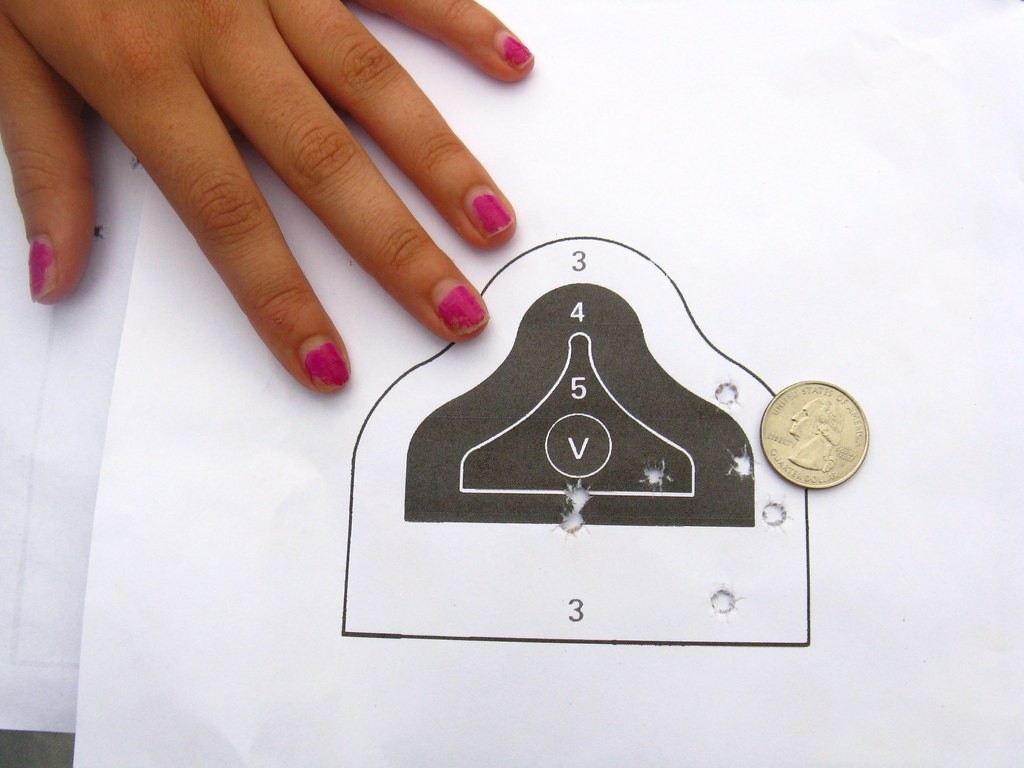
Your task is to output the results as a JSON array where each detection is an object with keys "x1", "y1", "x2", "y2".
[{"x1": 1, "y1": 0, "x2": 535, "y2": 394}]
